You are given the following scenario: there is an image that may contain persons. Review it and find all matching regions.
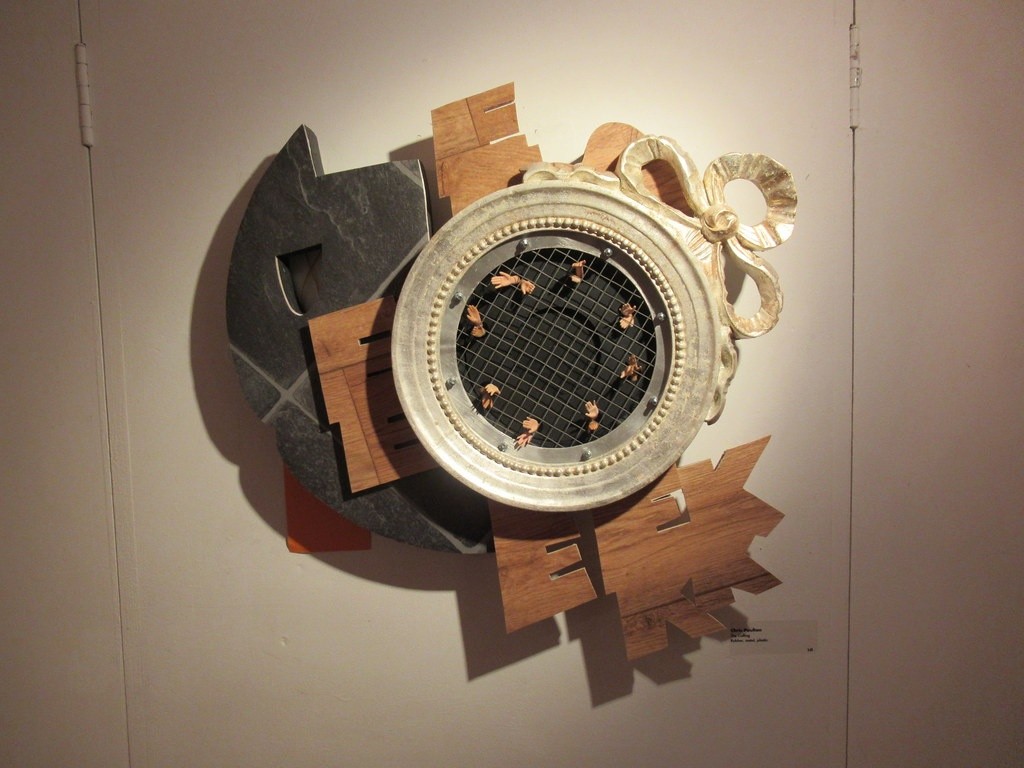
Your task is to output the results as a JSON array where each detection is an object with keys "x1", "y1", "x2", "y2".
[
  {"x1": 585, "y1": 400, "x2": 599, "y2": 419},
  {"x1": 466, "y1": 305, "x2": 486, "y2": 337},
  {"x1": 482, "y1": 384, "x2": 501, "y2": 408},
  {"x1": 516, "y1": 417, "x2": 539, "y2": 447},
  {"x1": 571, "y1": 260, "x2": 586, "y2": 283},
  {"x1": 491, "y1": 271, "x2": 535, "y2": 294},
  {"x1": 619, "y1": 303, "x2": 636, "y2": 329},
  {"x1": 620, "y1": 355, "x2": 640, "y2": 381}
]
